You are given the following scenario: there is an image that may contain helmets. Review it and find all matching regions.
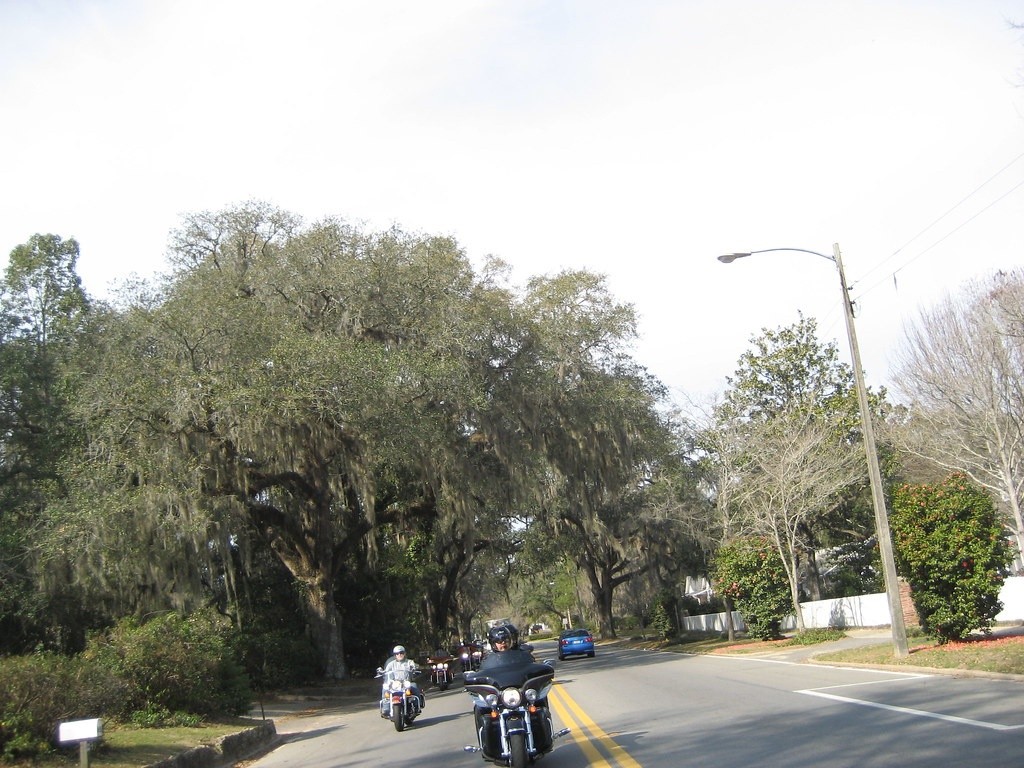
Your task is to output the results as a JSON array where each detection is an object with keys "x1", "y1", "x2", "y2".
[
  {"x1": 463, "y1": 639, "x2": 469, "y2": 644},
  {"x1": 393, "y1": 645, "x2": 406, "y2": 654},
  {"x1": 474, "y1": 639, "x2": 484, "y2": 647},
  {"x1": 507, "y1": 624, "x2": 519, "y2": 640},
  {"x1": 487, "y1": 627, "x2": 512, "y2": 639}
]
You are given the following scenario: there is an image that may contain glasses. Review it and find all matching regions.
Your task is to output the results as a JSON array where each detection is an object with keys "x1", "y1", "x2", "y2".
[{"x1": 494, "y1": 638, "x2": 511, "y2": 644}]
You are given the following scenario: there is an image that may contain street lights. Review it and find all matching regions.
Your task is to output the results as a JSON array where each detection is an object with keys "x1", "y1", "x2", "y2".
[{"x1": 715, "y1": 242, "x2": 911, "y2": 660}]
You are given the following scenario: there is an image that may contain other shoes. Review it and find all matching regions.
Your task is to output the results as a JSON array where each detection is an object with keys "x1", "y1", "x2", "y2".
[{"x1": 382, "y1": 707, "x2": 390, "y2": 716}]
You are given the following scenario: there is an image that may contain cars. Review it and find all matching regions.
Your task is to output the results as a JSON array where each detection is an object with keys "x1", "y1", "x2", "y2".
[{"x1": 554, "y1": 628, "x2": 595, "y2": 661}]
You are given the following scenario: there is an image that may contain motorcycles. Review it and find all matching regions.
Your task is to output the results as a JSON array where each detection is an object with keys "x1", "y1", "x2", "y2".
[
  {"x1": 458, "y1": 646, "x2": 482, "y2": 672},
  {"x1": 426, "y1": 654, "x2": 459, "y2": 692},
  {"x1": 374, "y1": 667, "x2": 426, "y2": 732},
  {"x1": 463, "y1": 650, "x2": 571, "y2": 768}
]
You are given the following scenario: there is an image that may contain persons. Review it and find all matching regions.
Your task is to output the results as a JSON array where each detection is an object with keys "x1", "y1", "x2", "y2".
[
  {"x1": 382, "y1": 645, "x2": 420, "y2": 714},
  {"x1": 427, "y1": 623, "x2": 534, "y2": 680}
]
